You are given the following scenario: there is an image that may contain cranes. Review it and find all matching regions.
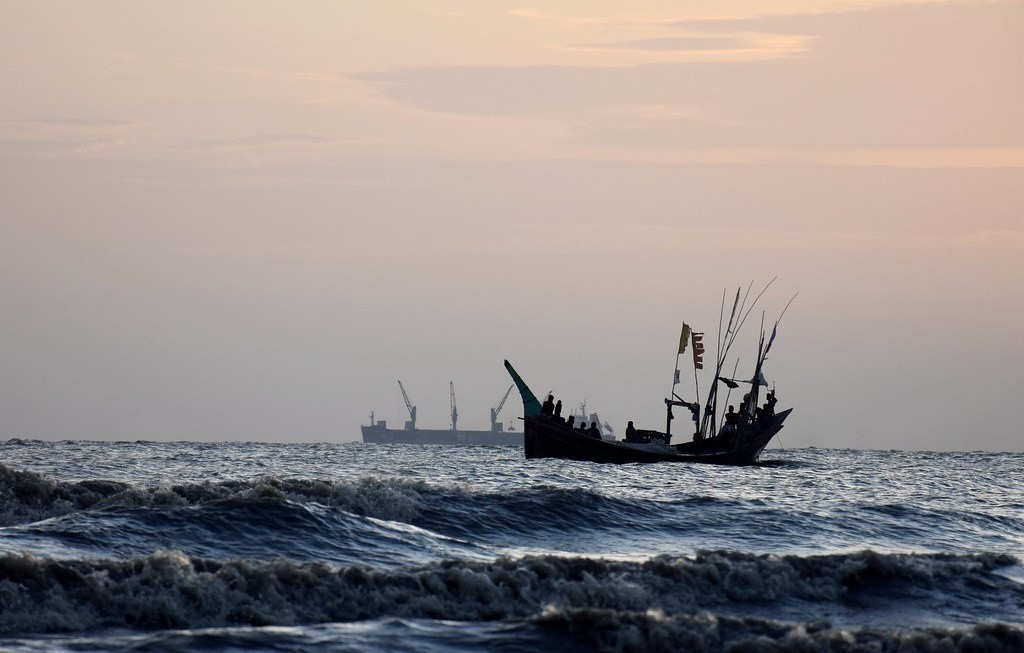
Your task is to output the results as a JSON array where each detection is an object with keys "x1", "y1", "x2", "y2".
[
  {"x1": 491, "y1": 384, "x2": 514, "y2": 434},
  {"x1": 450, "y1": 381, "x2": 459, "y2": 430},
  {"x1": 396, "y1": 379, "x2": 417, "y2": 429}
]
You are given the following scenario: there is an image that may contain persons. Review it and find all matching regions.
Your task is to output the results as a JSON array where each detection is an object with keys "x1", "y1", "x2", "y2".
[{"x1": 543, "y1": 393, "x2": 777, "y2": 443}]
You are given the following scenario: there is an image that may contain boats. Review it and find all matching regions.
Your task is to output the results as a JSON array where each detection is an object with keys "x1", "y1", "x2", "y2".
[
  {"x1": 361, "y1": 392, "x2": 616, "y2": 445},
  {"x1": 503, "y1": 276, "x2": 798, "y2": 465}
]
